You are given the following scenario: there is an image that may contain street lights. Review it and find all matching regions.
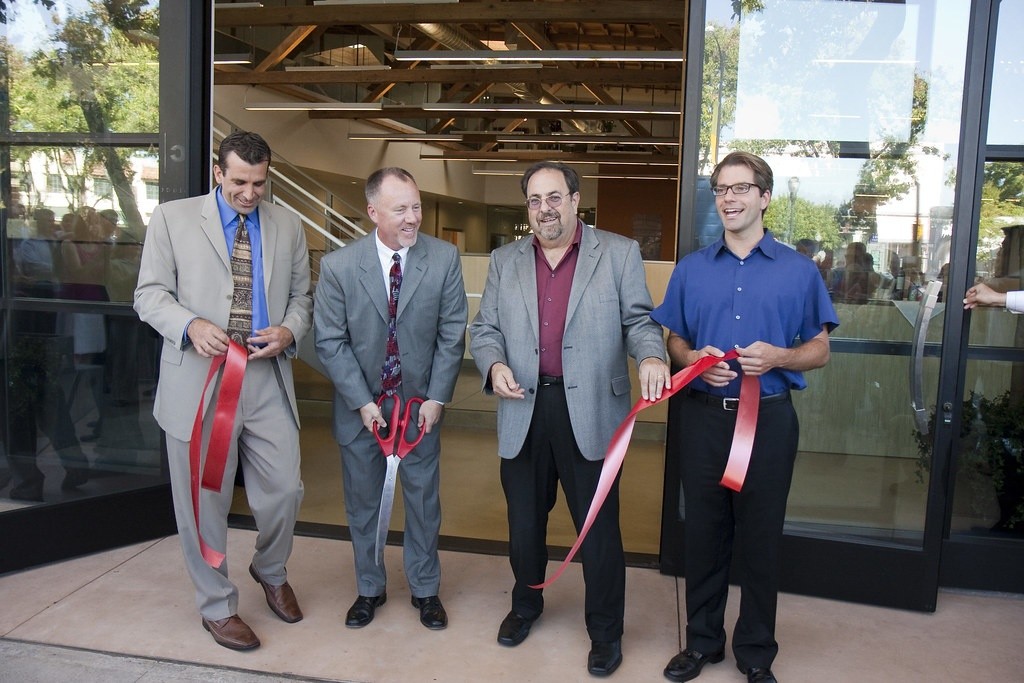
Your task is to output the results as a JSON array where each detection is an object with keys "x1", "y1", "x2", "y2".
[{"x1": 788, "y1": 177, "x2": 800, "y2": 244}]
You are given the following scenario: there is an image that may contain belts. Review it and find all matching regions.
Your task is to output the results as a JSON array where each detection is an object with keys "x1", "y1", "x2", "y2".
[
  {"x1": 682, "y1": 387, "x2": 789, "y2": 411},
  {"x1": 538, "y1": 376, "x2": 562, "y2": 386}
]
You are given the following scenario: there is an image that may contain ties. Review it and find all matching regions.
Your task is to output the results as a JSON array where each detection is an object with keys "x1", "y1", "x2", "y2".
[
  {"x1": 226, "y1": 213, "x2": 253, "y2": 355},
  {"x1": 380, "y1": 253, "x2": 402, "y2": 397}
]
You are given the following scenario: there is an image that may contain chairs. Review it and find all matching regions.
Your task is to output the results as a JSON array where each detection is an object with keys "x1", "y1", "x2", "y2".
[{"x1": 44, "y1": 282, "x2": 112, "y2": 303}]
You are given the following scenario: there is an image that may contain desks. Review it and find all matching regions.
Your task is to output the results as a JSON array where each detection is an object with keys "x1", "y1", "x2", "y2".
[{"x1": 11, "y1": 296, "x2": 152, "y2": 409}]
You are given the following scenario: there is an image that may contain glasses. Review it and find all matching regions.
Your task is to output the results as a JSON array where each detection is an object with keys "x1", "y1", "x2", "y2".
[
  {"x1": 524, "y1": 190, "x2": 574, "y2": 210},
  {"x1": 711, "y1": 182, "x2": 764, "y2": 195}
]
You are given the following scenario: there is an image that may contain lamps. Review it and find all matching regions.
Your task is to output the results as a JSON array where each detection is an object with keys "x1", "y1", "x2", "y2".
[{"x1": 209, "y1": 42, "x2": 689, "y2": 181}]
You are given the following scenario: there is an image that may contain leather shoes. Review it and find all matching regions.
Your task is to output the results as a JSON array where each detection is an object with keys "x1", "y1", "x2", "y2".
[
  {"x1": 663, "y1": 648, "x2": 725, "y2": 683},
  {"x1": 411, "y1": 595, "x2": 448, "y2": 629},
  {"x1": 497, "y1": 609, "x2": 542, "y2": 645},
  {"x1": 587, "y1": 638, "x2": 623, "y2": 675},
  {"x1": 345, "y1": 593, "x2": 387, "y2": 627},
  {"x1": 249, "y1": 563, "x2": 303, "y2": 623},
  {"x1": 736, "y1": 661, "x2": 778, "y2": 683},
  {"x1": 202, "y1": 613, "x2": 260, "y2": 649}
]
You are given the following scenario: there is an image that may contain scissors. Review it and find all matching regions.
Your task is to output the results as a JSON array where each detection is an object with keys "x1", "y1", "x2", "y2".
[{"x1": 373, "y1": 392, "x2": 425, "y2": 565}]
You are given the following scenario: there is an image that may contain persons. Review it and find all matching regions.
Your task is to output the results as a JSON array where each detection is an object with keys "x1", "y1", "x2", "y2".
[
  {"x1": 6, "y1": 201, "x2": 155, "y2": 502},
  {"x1": 961, "y1": 282, "x2": 1024, "y2": 317},
  {"x1": 469, "y1": 162, "x2": 670, "y2": 676},
  {"x1": 132, "y1": 132, "x2": 312, "y2": 652},
  {"x1": 648, "y1": 151, "x2": 840, "y2": 683},
  {"x1": 796, "y1": 237, "x2": 981, "y2": 304},
  {"x1": 314, "y1": 167, "x2": 468, "y2": 631}
]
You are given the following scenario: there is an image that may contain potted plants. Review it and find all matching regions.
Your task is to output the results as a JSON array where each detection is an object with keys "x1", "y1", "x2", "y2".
[{"x1": 912, "y1": 391, "x2": 1024, "y2": 536}]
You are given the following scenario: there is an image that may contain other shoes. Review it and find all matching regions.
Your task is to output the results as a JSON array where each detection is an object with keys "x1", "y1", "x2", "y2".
[
  {"x1": 10, "y1": 481, "x2": 50, "y2": 501},
  {"x1": 62, "y1": 464, "x2": 99, "y2": 491}
]
